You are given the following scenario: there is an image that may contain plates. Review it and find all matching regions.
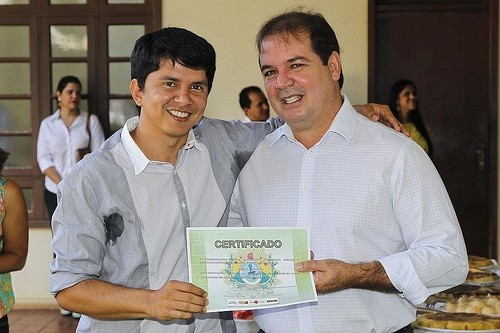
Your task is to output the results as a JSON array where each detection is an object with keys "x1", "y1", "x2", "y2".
[{"x1": 411, "y1": 258, "x2": 500, "y2": 333}]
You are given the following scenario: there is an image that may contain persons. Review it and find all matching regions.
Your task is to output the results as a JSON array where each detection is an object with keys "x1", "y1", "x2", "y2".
[
  {"x1": 0, "y1": 146, "x2": 31, "y2": 333},
  {"x1": 228, "y1": 7, "x2": 469, "y2": 332},
  {"x1": 48, "y1": 26, "x2": 413, "y2": 333},
  {"x1": 387, "y1": 76, "x2": 438, "y2": 166},
  {"x1": 236, "y1": 84, "x2": 274, "y2": 121},
  {"x1": 35, "y1": 74, "x2": 108, "y2": 318}
]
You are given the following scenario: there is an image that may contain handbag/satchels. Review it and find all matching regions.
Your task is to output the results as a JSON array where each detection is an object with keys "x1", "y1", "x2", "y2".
[{"x1": 76, "y1": 114, "x2": 91, "y2": 163}]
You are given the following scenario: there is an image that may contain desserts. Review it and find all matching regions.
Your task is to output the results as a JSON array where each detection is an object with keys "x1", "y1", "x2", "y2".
[
  {"x1": 468, "y1": 254, "x2": 500, "y2": 284},
  {"x1": 415, "y1": 293, "x2": 500, "y2": 330}
]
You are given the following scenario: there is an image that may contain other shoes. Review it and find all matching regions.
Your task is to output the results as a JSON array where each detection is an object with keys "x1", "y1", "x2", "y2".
[{"x1": 60, "y1": 309, "x2": 81, "y2": 318}]
